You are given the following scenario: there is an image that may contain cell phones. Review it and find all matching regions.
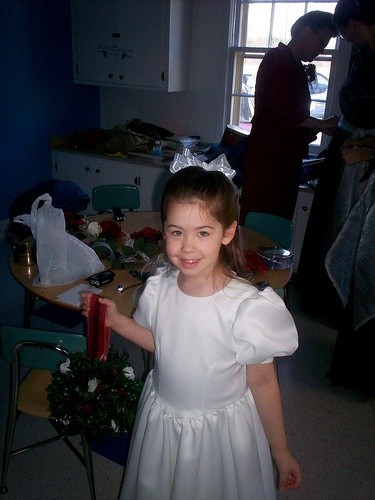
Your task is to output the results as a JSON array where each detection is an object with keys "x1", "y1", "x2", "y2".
[{"x1": 88, "y1": 271, "x2": 115, "y2": 289}]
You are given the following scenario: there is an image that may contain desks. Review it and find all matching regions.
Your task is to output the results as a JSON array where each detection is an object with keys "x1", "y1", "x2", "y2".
[{"x1": 8, "y1": 211, "x2": 294, "y2": 467}]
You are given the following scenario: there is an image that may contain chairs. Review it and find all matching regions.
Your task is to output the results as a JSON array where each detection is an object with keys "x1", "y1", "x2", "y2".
[
  {"x1": 244, "y1": 212, "x2": 293, "y2": 249},
  {"x1": 0, "y1": 326, "x2": 95, "y2": 500},
  {"x1": 91, "y1": 184, "x2": 140, "y2": 214}
]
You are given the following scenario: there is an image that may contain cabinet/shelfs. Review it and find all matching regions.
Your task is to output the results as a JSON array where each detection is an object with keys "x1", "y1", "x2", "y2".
[
  {"x1": 70, "y1": 0, "x2": 193, "y2": 92},
  {"x1": 51, "y1": 149, "x2": 173, "y2": 218}
]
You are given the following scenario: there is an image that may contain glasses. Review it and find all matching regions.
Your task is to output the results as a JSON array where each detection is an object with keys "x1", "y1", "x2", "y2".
[{"x1": 339, "y1": 27, "x2": 352, "y2": 40}]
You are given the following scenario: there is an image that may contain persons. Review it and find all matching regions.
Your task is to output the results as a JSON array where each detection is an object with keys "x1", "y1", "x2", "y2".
[
  {"x1": 81, "y1": 148, "x2": 302, "y2": 500},
  {"x1": 238, "y1": 0, "x2": 375, "y2": 334}
]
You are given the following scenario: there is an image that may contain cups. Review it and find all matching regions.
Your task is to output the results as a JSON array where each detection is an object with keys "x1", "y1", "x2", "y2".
[{"x1": 12, "y1": 242, "x2": 36, "y2": 266}]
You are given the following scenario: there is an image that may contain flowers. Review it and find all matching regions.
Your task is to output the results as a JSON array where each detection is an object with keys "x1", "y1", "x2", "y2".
[{"x1": 302, "y1": 63, "x2": 317, "y2": 82}]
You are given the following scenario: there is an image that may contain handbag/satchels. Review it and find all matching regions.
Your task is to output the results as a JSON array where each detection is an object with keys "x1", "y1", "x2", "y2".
[{"x1": 13, "y1": 193, "x2": 105, "y2": 287}]
